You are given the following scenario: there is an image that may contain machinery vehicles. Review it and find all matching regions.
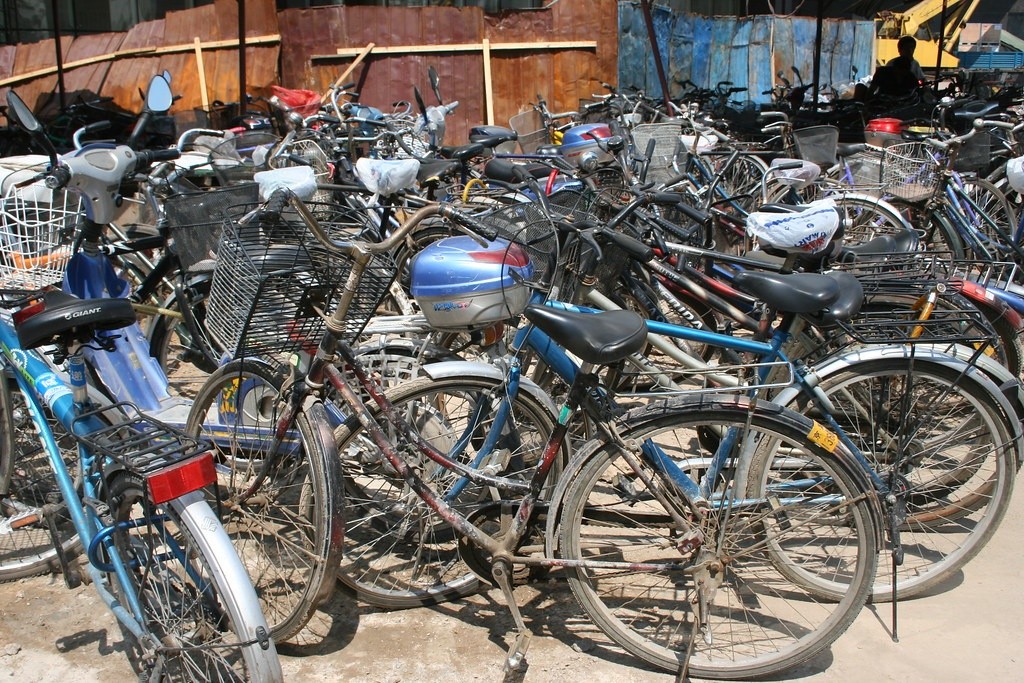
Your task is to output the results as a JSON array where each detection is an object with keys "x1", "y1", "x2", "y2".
[{"x1": 875, "y1": 0, "x2": 980, "y2": 69}]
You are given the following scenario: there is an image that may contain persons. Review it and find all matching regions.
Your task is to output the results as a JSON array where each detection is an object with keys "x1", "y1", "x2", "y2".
[{"x1": 884, "y1": 36, "x2": 955, "y2": 102}]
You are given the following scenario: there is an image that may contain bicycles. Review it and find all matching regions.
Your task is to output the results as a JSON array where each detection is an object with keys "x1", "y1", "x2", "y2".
[{"x1": 1, "y1": 64, "x2": 1023, "y2": 683}]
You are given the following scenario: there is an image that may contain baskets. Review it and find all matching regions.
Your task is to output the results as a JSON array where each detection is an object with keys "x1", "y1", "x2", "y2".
[
  {"x1": 882, "y1": 142, "x2": 939, "y2": 201},
  {"x1": 480, "y1": 190, "x2": 640, "y2": 304},
  {"x1": 0, "y1": 183, "x2": 83, "y2": 290},
  {"x1": 161, "y1": 132, "x2": 399, "y2": 359},
  {"x1": 382, "y1": 113, "x2": 427, "y2": 159},
  {"x1": 508, "y1": 110, "x2": 551, "y2": 155},
  {"x1": 952, "y1": 133, "x2": 991, "y2": 172},
  {"x1": 831, "y1": 79, "x2": 855, "y2": 99},
  {"x1": 631, "y1": 123, "x2": 682, "y2": 168},
  {"x1": 791, "y1": 125, "x2": 839, "y2": 165}
]
